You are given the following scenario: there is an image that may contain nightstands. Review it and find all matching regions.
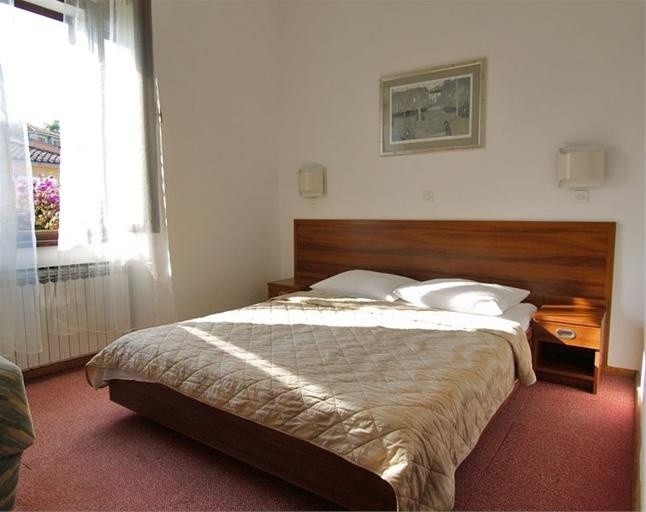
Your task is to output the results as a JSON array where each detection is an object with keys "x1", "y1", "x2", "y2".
[
  {"x1": 533, "y1": 303, "x2": 607, "y2": 395},
  {"x1": 267, "y1": 275, "x2": 323, "y2": 299}
]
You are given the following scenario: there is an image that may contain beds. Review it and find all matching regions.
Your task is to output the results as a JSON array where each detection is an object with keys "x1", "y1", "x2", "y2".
[{"x1": 107, "y1": 288, "x2": 538, "y2": 512}]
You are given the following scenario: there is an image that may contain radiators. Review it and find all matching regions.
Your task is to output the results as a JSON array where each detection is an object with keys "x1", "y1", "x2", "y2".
[{"x1": 0, "y1": 259, "x2": 131, "y2": 372}]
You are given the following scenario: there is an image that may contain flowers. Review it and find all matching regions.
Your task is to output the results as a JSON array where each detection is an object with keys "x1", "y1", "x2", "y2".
[{"x1": 12, "y1": 172, "x2": 61, "y2": 231}]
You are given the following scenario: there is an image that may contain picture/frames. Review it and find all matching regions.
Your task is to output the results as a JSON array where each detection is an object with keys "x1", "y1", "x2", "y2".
[{"x1": 378, "y1": 57, "x2": 487, "y2": 158}]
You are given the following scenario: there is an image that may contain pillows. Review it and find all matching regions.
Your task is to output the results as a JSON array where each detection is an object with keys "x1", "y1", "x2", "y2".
[{"x1": 309, "y1": 269, "x2": 532, "y2": 318}]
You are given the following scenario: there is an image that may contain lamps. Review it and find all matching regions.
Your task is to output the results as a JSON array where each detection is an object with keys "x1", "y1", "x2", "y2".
[
  {"x1": 558, "y1": 143, "x2": 606, "y2": 188},
  {"x1": 297, "y1": 167, "x2": 325, "y2": 198}
]
